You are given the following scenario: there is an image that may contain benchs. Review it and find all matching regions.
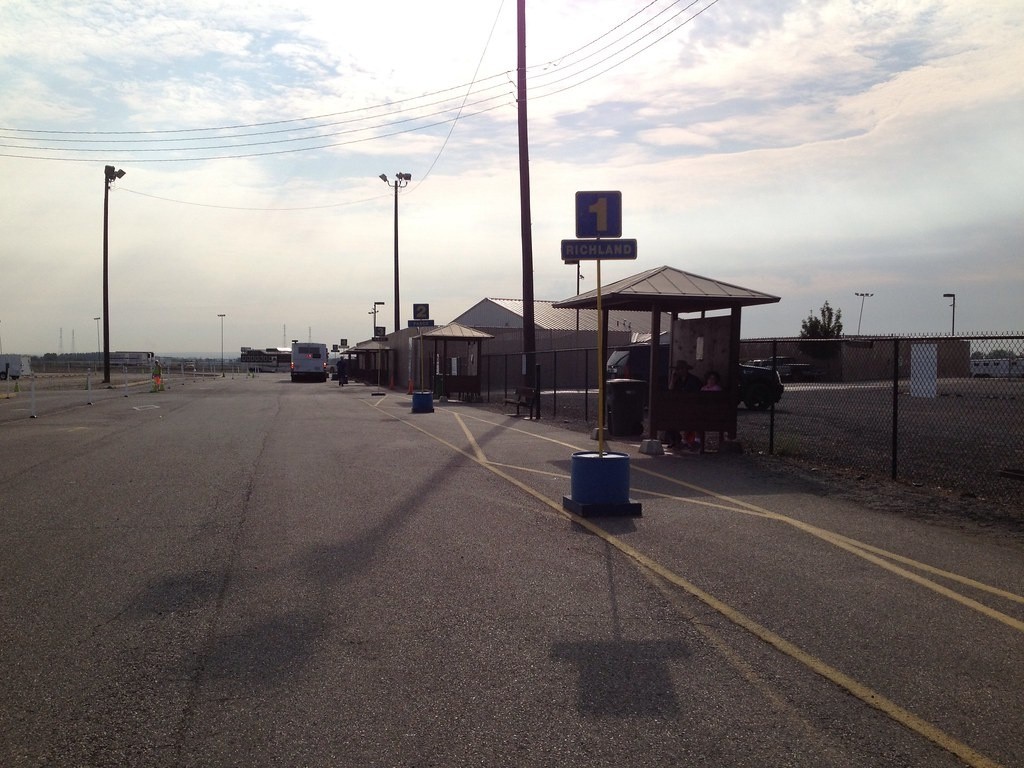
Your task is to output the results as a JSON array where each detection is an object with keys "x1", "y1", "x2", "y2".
[{"x1": 502, "y1": 387, "x2": 536, "y2": 419}]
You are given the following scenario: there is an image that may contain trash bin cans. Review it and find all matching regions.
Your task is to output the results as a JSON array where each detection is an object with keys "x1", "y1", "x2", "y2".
[
  {"x1": 436, "y1": 375, "x2": 450, "y2": 400},
  {"x1": 605, "y1": 379, "x2": 647, "y2": 437}
]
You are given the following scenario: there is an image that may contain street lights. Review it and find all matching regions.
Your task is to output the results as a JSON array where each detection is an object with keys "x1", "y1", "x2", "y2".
[
  {"x1": 855, "y1": 292, "x2": 873, "y2": 335},
  {"x1": 217, "y1": 314, "x2": 226, "y2": 377},
  {"x1": 93, "y1": 317, "x2": 101, "y2": 367},
  {"x1": 378, "y1": 172, "x2": 413, "y2": 331},
  {"x1": 368, "y1": 302, "x2": 385, "y2": 384},
  {"x1": 100, "y1": 164, "x2": 126, "y2": 384},
  {"x1": 942, "y1": 292, "x2": 957, "y2": 337},
  {"x1": 565, "y1": 258, "x2": 581, "y2": 331}
]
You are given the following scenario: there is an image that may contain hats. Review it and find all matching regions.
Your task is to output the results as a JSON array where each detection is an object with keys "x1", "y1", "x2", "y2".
[{"x1": 668, "y1": 360, "x2": 693, "y2": 369}]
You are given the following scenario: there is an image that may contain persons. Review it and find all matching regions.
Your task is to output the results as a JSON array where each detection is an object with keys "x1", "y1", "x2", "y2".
[
  {"x1": 152, "y1": 360, "x2": 162, "y2": 391},
  {"x1": 666, "y1": 359, "x2": 722, "y2": 449}
]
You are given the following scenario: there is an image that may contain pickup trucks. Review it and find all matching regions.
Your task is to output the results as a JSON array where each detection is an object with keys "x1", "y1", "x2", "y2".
[{"x1": 744, "y1": 353, "x2": 812, "y2": 382}]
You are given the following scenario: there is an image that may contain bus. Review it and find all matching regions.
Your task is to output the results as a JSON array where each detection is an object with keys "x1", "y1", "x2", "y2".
[{"x1": 290, "y1": 343, "x2": 329, "y2": 382}]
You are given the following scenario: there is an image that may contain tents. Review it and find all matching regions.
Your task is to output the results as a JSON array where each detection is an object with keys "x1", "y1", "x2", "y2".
[
  {"x1": 412, "y1": 322, "x2": 496, "y2": 406},
  {"x1": 340, "y1": 342, "x2": 394, "y2": 386},
  {"x1": 552, "y1": 266, "x2": 781, "y2": 455}
]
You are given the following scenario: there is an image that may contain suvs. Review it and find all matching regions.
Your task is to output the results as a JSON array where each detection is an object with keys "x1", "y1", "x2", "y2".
[{"x1": 607, "y1": 342, "x2": 785, "y2": 413}]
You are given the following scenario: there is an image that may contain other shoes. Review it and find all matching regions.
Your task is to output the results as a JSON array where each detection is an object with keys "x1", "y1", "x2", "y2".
[
  {"x1": 668, "y1": 441, "x2": 675, "y2": 447},
  {"x1": 689, "y1": 442, "x2": 700, "y2": 451},
  {"x1": 678, "y1": 442, "x2": 688, "y2": 450}
]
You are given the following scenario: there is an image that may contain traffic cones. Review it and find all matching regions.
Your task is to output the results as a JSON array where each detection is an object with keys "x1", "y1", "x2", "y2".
[
  {"x1": 159, "y1": 377, "x2": 166, "y2": 392},
  {"x1": 388, "y1": 375, "x2": 396, "y2": 390},
  {"x1": 13, "y1": 379, "x2": 20, "y2": 392},
  {"x1": 405, "y1": 378, "x2": 415, "y2": 395},
  {"x1": 252, "y1": 372, "x2": 255, "y2": 378},
  {"x1": 149, "y1": 379, "x2": 157, "y2": 393},
  {"x1": 84, "y1": 377, "x2": 88, "y2": 390}
]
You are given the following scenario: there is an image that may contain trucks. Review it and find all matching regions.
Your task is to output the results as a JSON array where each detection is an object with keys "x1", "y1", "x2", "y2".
[{"x1": 0, "y1": 353, "x2": 32, "y2": 381}]
